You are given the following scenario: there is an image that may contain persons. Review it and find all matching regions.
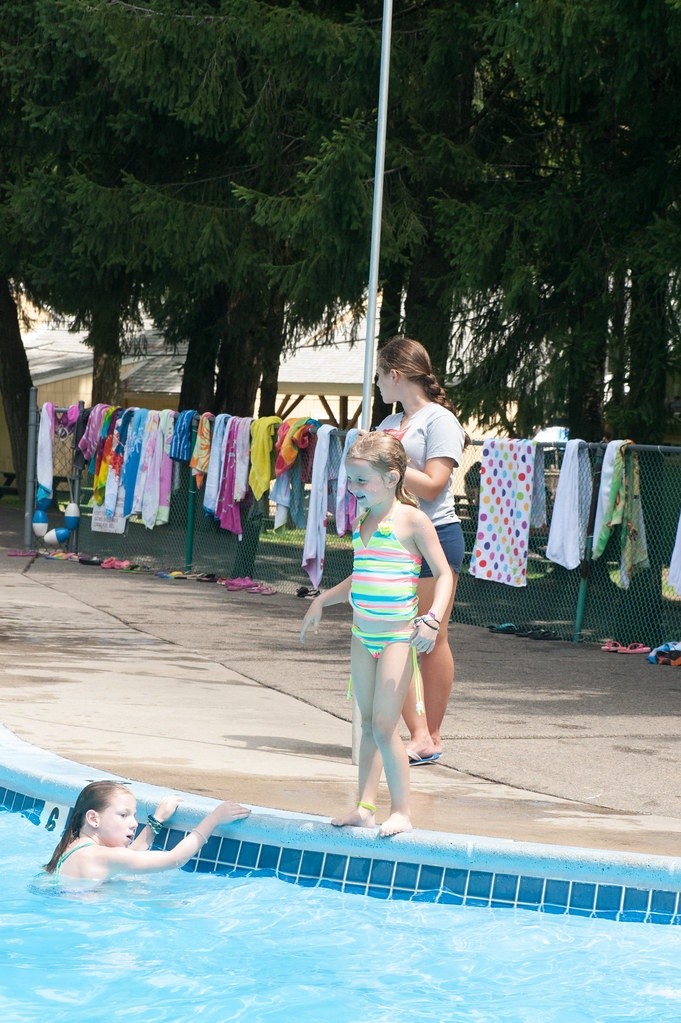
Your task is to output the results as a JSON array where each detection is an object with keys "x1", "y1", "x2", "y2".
[
  {"x1": 298, "y1": 431, "x2": 453, "y2": 836},
  {"x1": 370, "y1": 340, "x2": 466, "y2": 765},
  {"x1": 40, "y1": 778, "x2": 251, "y2": 900}
]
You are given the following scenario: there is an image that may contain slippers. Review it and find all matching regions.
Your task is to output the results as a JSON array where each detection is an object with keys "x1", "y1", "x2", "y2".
[
  {"x1": 617, "y1": 643, "x2": 651, "y2": 653},
  {"x1": 601, "y1": 640, "x2": 627, "y2": 651},
  {"x1": 405, "y1": 749, "x2": 440, "y2": 767},
  {"x1": 489, "y1": 623, "x2": 564, "y2": 643},
  {"x1": 5, "y1": 549, "x2": 276, "y2": 596}
]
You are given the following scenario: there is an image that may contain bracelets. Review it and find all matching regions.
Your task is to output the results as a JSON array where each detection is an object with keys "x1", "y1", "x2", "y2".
[
  {"x1": 433, "y1": 618, "x2": 439, "y2": 623},
  {"x1": 192, "y1": 829, "x2": 208, "y2": 844},
  {"x1": 422, "y1": 618, "x2": 437, "y2": 630},
  {"x1": 147, "y1": 814, "x2": 162, "y2": 835}
]
